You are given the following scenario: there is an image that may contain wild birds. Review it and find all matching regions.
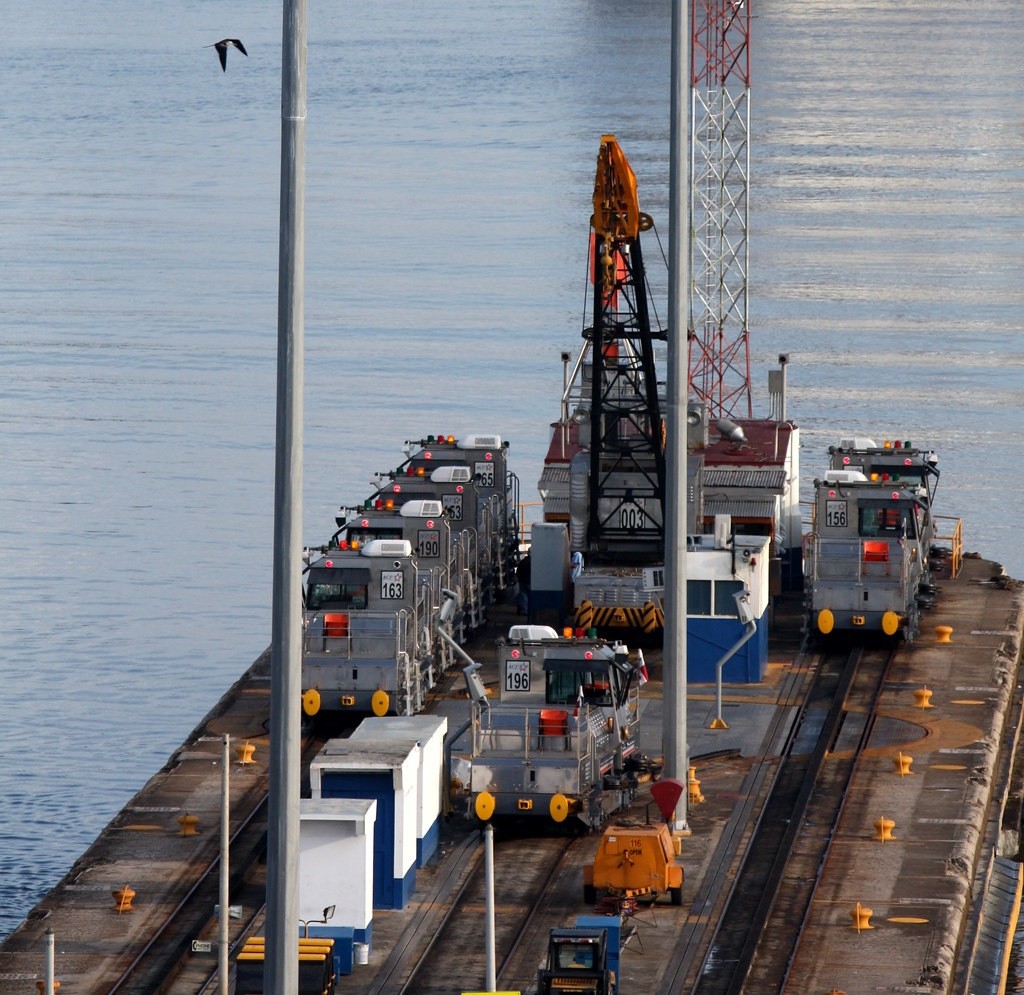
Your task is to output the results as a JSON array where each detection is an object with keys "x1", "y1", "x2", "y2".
[{"x1": 202, "y1": 38, "x2": 249, "y2": 73}]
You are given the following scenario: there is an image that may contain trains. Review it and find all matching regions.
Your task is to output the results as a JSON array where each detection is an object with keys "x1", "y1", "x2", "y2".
[
  {"x1": 806, "y1": 440, "x2": 939, "y2": 647},
  {"x1": 460, "y1": 620, "x2": 649, "y2": 828},
  {"x1": 301, "y1": 433, "x2": 518, "y2": 717}
]
